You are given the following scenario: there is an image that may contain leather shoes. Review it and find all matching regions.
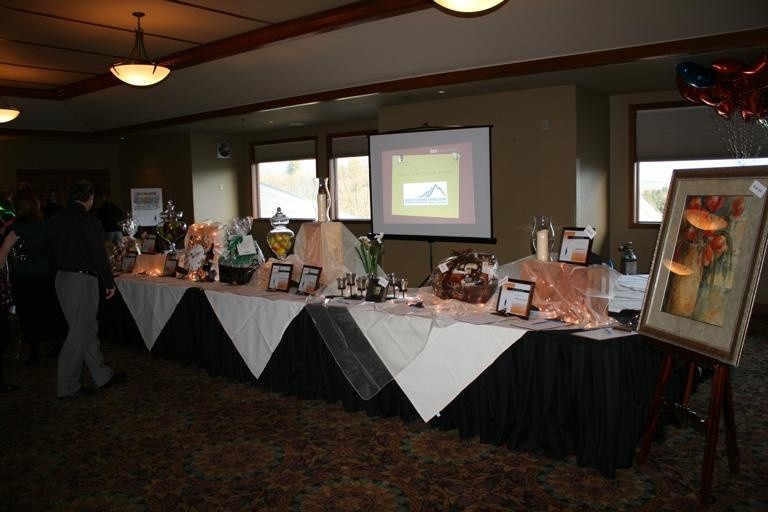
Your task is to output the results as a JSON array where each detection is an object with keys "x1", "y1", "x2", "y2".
[
  {"x1": 77, "y1": 387, "x2": 96, "y2": 397},
  {"x1": 102, "y1": 373, "x2": 128, "y2": 388}
]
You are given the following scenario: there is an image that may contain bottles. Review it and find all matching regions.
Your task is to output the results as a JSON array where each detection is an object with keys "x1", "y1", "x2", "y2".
[
  {"x1": 266, "y1": 207, "x2": 295, "y2": 260},
  {"x1": 620, "y1": 249, "x2": 639, "y2": 275},
  {"x1": 156, "y1": 199, "x2": 188, "y2": 254},
  {"x1": 531, "y1": 214, "x2": 555, "y2": 254}
]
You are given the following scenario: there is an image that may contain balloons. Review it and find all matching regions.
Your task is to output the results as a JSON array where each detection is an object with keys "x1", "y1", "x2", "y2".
[{"x1": 676, "y1": 52, "x2": 768, "y2": 121}]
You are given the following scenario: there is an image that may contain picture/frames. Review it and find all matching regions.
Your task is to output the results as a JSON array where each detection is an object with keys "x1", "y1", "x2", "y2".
[
  {"x1": 297, "y1": 264, "x2": 322, "y2": 296},
  {"x1": 495, "y1": 277, "x2": 536, "y2": 320},
  {"x1": 557, "y1": 226, "x2": 596, "y2": 267},
  {"x1": 267, "y1": 263, "x2": 294, "y2": 292},
  {"x1": 636, "y1": 165, "x2": 768, "y2": 368}
]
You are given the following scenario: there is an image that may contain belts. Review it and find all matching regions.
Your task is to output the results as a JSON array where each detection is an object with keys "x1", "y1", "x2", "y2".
[{"x1": 58, "y1": 267, "x2": 99, "y2": 277}]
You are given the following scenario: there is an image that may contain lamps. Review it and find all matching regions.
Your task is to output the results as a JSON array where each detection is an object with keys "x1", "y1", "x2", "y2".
[
  {"x1": 432, "y1": 0, "x2": 506, "y2": 17},
  {"x1": 0, "y1": 101, "x2": 21, "y2": 124},
  {"x1": 108, "y1": 11, "x2": 170, "y2": 88}
]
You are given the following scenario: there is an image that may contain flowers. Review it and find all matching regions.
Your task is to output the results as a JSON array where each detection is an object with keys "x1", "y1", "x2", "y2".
[{"x1": 354, "y1": 232, "x2": 386, "y2": 278}]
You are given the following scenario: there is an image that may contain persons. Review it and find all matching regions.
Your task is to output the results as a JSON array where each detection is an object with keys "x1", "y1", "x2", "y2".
[{"x1": 0, "y1": 181, "x2": 124, "y2": 404}]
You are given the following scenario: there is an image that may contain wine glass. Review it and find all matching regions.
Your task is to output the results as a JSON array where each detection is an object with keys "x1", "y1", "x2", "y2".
[
  {"x1": 388, "y1": 272, "x2": 397, "y2": 300},
  {"x1": 346, "y1": 272, "x2": 356, "y2": 297},
  {"x1": 357, "y1": 275, "x2": 368, "y2": 299},
  {"x1": 337, "y1": 277, "x2": 346, "y2": 297},
  {"x1": 398, "y1": 276, "x2": 409, "y2": 300}
]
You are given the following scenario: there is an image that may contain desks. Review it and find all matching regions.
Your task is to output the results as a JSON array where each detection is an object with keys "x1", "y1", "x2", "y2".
[{"x1": 100, "y1": 273, "x2": 703, "y2": 479}]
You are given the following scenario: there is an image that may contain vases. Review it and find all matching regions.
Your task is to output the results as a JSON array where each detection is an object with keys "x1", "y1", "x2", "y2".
[{"x1": 366, "y1": 278, "x2": 380, "y2": 301}]
